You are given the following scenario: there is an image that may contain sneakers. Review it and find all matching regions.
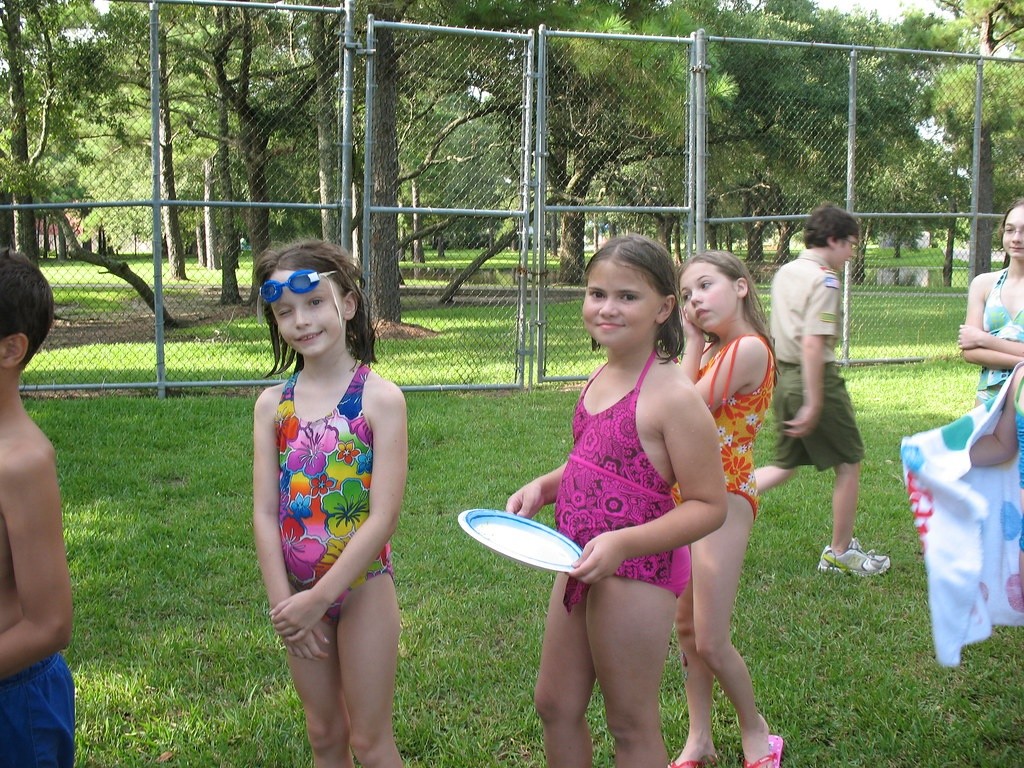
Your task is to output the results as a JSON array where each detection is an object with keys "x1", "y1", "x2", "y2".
[{"x1": 818, "y1": 538, "x2": 891, "y2": 577}]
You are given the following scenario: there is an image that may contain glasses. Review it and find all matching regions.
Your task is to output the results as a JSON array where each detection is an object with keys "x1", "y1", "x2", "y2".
[
  {"x1": 843, "y1": 237, "x2": 859, "y2": 251},
  {"x1": 1004, "y1": 226, "x2": 1024, "y2": 235},
  {"x1": 261, "y1": 269, "x2": 338, "y2": 303}
]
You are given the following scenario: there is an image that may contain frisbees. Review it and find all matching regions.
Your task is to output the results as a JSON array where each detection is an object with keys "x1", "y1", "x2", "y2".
[{"x1": 458, "y1": 509, "x2": 583, "y2": 574}]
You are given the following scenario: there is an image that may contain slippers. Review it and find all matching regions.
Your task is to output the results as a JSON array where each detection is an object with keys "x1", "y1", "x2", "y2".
[
  {"x1": 668, "y1": 753, "x2": 718, "y2": 768},
  {"x1": 744, "y1": 735, "x2": 784, "y2": 768}
]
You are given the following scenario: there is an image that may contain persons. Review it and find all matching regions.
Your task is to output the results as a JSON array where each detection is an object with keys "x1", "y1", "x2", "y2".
[
  {"x1": 0, "y1": 248, "x2": 76, "y2": 768},
  {"x1": 246, "y1": 240, "x2": 407, "y2": 767},
  {"x1": 668, "y1": 248, "x2": 783, "y2": 768},
  {"x1": 955, "y1": 199, "x2": 1024, "y2": 409},
  {"x1": 753, "y1": 201, "x2": 890, "y2": 577},
  {"x1": 902, "y1": 366, "x2": 1024, "y2": 606},
  {"x1": 505, "y1": 234, "x2": 728, "y2": 768}
]
